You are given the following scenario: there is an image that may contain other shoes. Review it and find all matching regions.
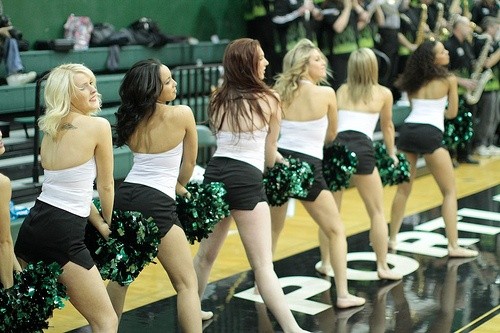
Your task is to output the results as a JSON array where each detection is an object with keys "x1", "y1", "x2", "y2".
[
  {"x1": 6, "y1": 71, "x2": 36, "y2": 87},
  {"x1": 475, "y1": 144, "x2": 491, "y2": 158},
  {"x1": 487, "y1": 144, "x2": 500, "y2": 156},
  {"x1": 456, "y1": 150, "x2": 479, "y2": 164}
]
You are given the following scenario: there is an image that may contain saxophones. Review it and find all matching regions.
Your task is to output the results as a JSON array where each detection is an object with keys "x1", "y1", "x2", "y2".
[
  {"x1": 463, "y1": 32, "x2": 494, "y2": 106},
  {"x1": 409, "y1": 0, "x2": 477, "y2": 45}
]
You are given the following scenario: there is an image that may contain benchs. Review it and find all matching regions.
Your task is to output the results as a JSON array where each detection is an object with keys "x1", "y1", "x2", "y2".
[{"x1": 0, "y1": 40, "x2": 411, "y2": 248}]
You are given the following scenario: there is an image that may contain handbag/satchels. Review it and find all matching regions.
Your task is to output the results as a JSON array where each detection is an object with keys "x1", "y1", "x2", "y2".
[{"x1": 63, "y1": 15, "x2": 93, "y2": 52}]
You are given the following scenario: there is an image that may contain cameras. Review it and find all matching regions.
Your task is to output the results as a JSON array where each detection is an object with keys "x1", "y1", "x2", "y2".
[{"x1": 0, "y1": 15, "x2": 23, "y2": 42}]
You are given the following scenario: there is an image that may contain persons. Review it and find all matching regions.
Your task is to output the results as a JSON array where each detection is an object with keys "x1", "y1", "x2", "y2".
[
  {"x1": 472, "y1": 16, "x2": 500, "y2": 158},
  {"x1": 107, "y1": 58, "x2": 202, "y2": 333},
  {"x1": 443, "y1": 17, "x2": 491, "y2": 168},
  {"x1": 315, "y1": 48, "x2": 403, "y2": 280},
  {"x1": 202, "y1": 233, "x2": 500, "y2": 333},
  {"x1": 254, "y1": 39, "x2": 366, "y2": 308},
  {"x1": 0, "y1": 5, "x2": 37, "y2": 87},
  {"x1": 194, "y1": 38, "x2": 311, "y2": 333},
  {"x1": 0, "y1": 132, "x2": 23, "y2": 290},
  {"x1": 369, "y1": 36, "x2": 478, "y2": 256},
  {"x1": 14, "y1": 63, "x2": 119, "y2": 333},
  {"x1": 238, "y1": 0, "x2": 500, "y2": 107}
]
